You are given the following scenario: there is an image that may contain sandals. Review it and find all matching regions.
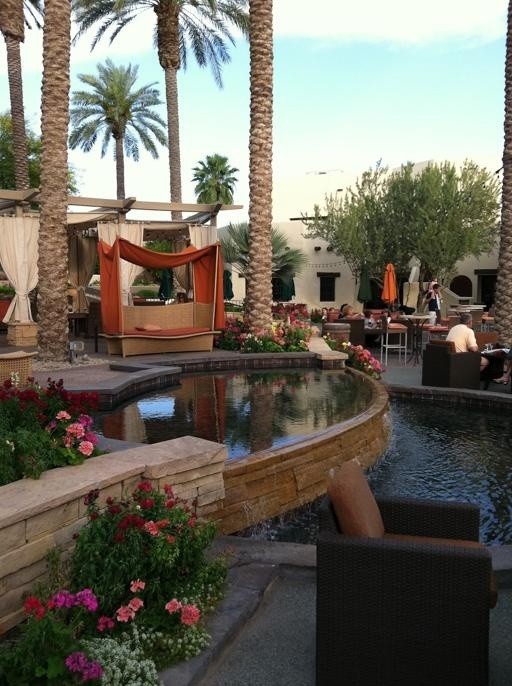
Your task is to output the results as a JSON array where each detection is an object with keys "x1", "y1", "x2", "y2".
[{"x1": 494, "y1": 378, "x2": 509, "y2": 384}]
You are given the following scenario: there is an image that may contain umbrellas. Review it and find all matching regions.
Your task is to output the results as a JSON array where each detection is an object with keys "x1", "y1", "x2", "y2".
[{"x1": 380, "y1": 262, "x2": 398, "y2": 308}]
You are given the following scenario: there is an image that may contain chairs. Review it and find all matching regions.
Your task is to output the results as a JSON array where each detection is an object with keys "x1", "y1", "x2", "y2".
[{"x1": 421, "y1": 331, "x2": 512, "y2": 390}]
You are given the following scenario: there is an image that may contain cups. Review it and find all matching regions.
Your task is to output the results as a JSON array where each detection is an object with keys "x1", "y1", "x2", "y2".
[{"x1": 487, "y1": 343, "x2": 492, "y2": 351}]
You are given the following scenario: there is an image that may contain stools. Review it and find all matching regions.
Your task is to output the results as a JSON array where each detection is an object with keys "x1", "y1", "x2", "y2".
[
  {"x1": 415, "y1": 323, "x2": 449, "y2": 346},
  {"x1": 380, "y1": 323, "x2": 408, "y2": 368}
]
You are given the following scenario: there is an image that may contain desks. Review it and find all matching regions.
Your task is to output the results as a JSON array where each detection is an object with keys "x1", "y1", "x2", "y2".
[{"x1": 397, "y1": 314, "x2": 433, "y2": 370}]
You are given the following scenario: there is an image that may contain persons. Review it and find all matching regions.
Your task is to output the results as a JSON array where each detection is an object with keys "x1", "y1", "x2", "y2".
[
  {"x1": 178, "y1": 293, "x2": 188, "y2": 304},
  {"x1": 374, "y1": 310, "x2": 391, "y2": 342},
  {"x1": 175, "y1": 292, "x2": 183, "y2": 304},
  {"x1": 364, "y1": 310, "x2": 377, "y2": 329},
  {"x1": 446, "y1": 313, "x2": 489, "y2": 373},
  {"x1": 424, "y1": 284, "x2": 443, "y2": 336},
  {"x1": 321, "y1": 308, "x2": 328, "y2": 319},
  {"x1": 428, "y1": 277, "x2": 443, "y2": 301},
  {"x1": 329, "y1": 307, "x2": 335, "y2": 312},
  {"x1": 342, "y1": 305, "x2": 361, "y2": 319},
  {"x1": 338, "y1": 303, "x2": 348, "y2": 318},
  {"x1": 491, "y1": 348, "x2": 512, "y2": 385}
]
